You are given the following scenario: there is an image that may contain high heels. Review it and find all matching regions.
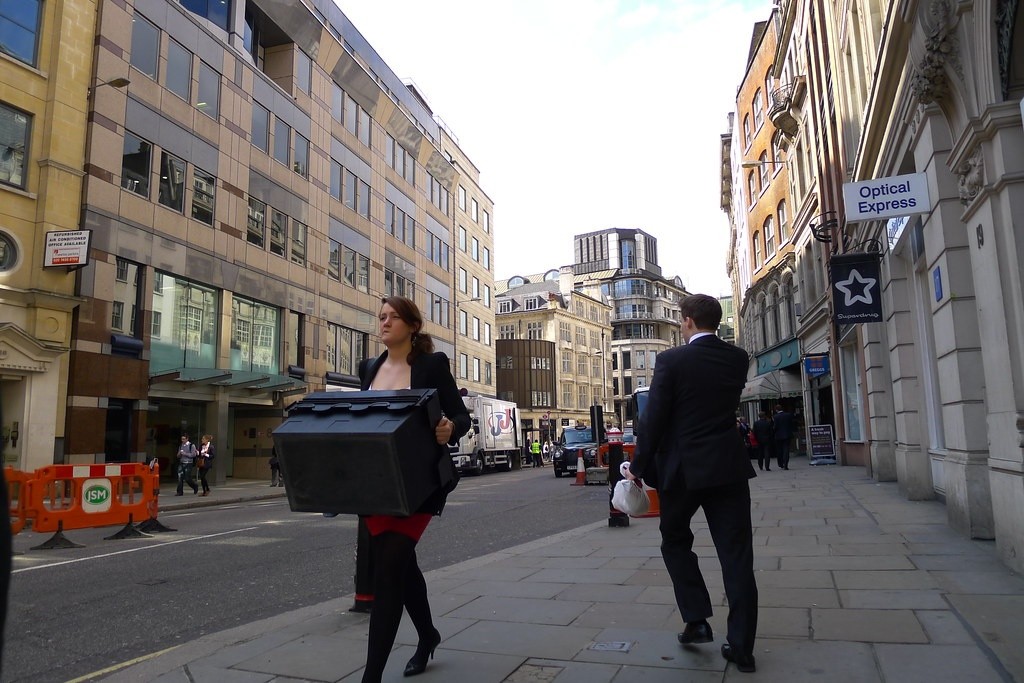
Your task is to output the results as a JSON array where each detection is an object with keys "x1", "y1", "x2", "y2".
[{"x1": 403, "y1": 628, "x2": 441, "y2": 677}]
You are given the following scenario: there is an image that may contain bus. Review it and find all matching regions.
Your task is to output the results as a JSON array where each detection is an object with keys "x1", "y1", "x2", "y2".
[{"x1": 630, "y1": 386, "x2": 651, "y2": 447}]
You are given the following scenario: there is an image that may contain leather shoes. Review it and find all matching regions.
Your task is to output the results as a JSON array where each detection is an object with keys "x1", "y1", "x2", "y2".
[
  {"x1": 678, "y1": 623, "x2": 713, "y2": 644},
  {"x1": 722, "y1": 643, "x2": 755, "y2": 672}
]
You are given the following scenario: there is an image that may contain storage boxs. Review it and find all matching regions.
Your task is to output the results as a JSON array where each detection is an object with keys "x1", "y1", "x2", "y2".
[{"x1": 272, "y1": 387, "x2": 453, "y2": 515}]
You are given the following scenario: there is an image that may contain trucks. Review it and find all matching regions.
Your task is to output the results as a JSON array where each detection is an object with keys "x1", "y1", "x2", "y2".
[{"x1": 440, "y1": 395, "x2": 522, "y2": 477}]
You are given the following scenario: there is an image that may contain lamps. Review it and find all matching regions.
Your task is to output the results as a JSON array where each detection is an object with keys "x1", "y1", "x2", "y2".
[
  {"x1": 742, "y1": 159, "x2": 785, "y2": 170},
  {"x1": 88, "y1": 77, "x2": 130, "y2": 99},
  {"x1": 456, "y1": 297, "x2": 481, "y2": 306}
]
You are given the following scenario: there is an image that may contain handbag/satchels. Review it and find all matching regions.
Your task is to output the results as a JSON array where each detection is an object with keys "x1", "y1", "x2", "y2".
[
  {"x1": 611, "y1": 462, "x2": 650, "y2": 515},
  {"x1": 195, "y1": 451, "x2": 207, "y2": 468}
]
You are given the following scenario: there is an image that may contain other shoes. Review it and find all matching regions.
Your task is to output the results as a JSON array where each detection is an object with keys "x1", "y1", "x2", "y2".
[
  {"x1": 270, "y1": 484, "x2": 277, "y2": 487},
  {"x1": 194, "y1": 485, "x2": 198, "y2": 494},
  {"x1": 202, "y1": 490, "x2": 211, "y2": 496},
  {"x1": 278, "y1": 483, "x2": 283, "y2": 487},
  {"x1": 175, "y1": 493, "x2": 183, "y2": 496}
]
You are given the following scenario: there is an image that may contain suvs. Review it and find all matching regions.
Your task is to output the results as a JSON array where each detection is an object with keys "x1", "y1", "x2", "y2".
[{"x1": 551, "y1": 425, "x2": 596, "y2": 478}]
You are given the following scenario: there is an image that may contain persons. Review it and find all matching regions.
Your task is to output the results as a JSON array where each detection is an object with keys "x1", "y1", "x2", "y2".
[
  {"x1": 196, "y1": 435, "x2": 215, "y2": 496},
  {"x1": 735, "y1": 410, "x2": 759, "y2": 451},
  {"x1": 753, "y1": 412, "x2": 771, "y2": 471},
  {"x1": 630, "y1": 294, "x2": 760, "y2": 676},
  {"x1": 357, "y1": 295, "x2": 473, "y2": 683},
  {"x1": 772, "y1": 404, "x2": 792, "y2": 471},
  {"x1": 531, "y1": 440, "x2": 540, "y2": 467},
  {"x1": 175, "y1": 433, "x2": 199, "y2": 496}
]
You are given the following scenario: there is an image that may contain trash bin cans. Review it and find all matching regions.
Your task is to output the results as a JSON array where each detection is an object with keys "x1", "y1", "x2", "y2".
[{"x1": 624, "y1": 444, "x2": 661, "y2": 518}]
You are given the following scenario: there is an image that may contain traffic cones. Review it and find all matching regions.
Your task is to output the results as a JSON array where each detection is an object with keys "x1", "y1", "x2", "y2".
[
  {"x1": 570, "y1": 449, "x2": 588, "y2": 486},
  {"x1": 629, "y1": 477, "x2": 659, "y2": 519}
]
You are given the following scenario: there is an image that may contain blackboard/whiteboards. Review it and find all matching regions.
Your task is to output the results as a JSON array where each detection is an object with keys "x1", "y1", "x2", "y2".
[{"x1": 808, "y1": 424, "x2": 835, "y2": 457}]
